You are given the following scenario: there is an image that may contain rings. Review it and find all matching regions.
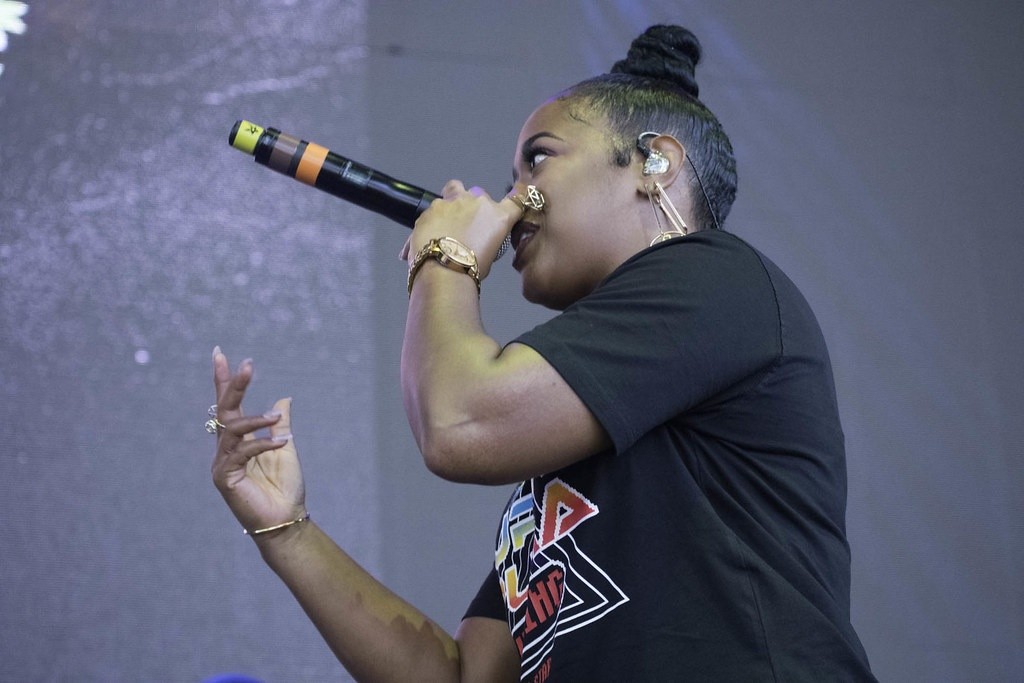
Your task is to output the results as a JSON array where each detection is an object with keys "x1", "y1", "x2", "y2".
[
  {"x1": 205, "y1": 404, "x2": 230, "y2": 435},
  {"x1": 504, "y1": 185, "x2": 546, "y2": 221}
]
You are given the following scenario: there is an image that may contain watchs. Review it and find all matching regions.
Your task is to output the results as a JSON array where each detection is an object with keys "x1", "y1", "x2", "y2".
[{"x1": 407, "y1": 237, "x2": 481, "y2": 302}]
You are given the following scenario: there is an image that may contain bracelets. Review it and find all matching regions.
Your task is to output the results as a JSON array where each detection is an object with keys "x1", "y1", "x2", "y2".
[{"x1": 242, "y1": 510, "x2": 310, "y2": 535}]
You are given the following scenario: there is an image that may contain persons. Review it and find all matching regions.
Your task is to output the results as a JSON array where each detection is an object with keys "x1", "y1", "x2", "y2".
[{"x1": 210, "y1": 20, "x2": 878, "y2": 683}]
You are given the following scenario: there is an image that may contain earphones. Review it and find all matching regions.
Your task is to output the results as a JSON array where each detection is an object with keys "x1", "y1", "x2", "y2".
[{"x1": 643, "y1": 154, "x2": 669, "y2": 176}]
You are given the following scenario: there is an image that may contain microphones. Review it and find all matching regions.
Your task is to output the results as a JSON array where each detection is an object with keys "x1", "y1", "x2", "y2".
[{"x1": 229, "y1": 120, "x2": 512, "y2": 260}]
